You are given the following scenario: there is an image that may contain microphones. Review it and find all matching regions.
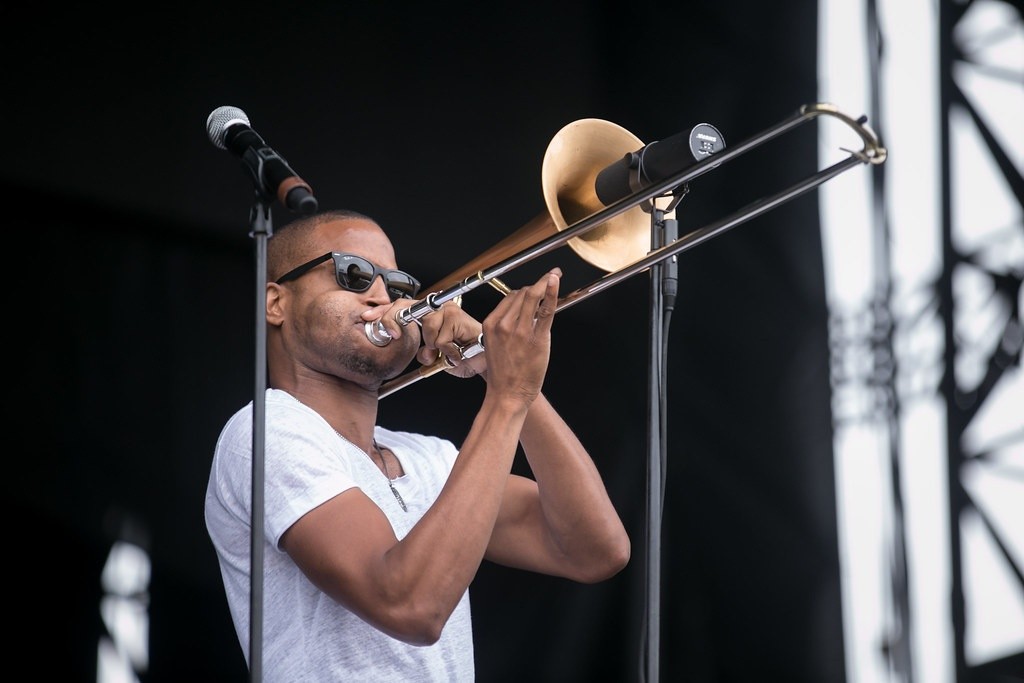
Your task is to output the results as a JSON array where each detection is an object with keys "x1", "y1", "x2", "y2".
[
  {"x1": 206, "y1": 106, "x2": 318, "y2": 215},
  {"x1": 595, "y1": 124, "x2": 727, "y2": 207}
]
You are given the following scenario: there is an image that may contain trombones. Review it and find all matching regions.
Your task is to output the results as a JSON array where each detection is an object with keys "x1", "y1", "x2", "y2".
[{"x1": 378, "y1": 102, "x2": 888, "y2": 399}]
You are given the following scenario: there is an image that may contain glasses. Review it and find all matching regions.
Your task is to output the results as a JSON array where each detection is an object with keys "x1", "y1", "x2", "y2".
[{"x1": 275, "y1": 252, "x2": 422, "y2": 304}]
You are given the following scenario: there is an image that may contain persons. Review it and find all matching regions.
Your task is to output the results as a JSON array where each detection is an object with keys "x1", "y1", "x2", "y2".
[{"x1": 204, "y1": 208, "x2": 630, "y2": 683}]
